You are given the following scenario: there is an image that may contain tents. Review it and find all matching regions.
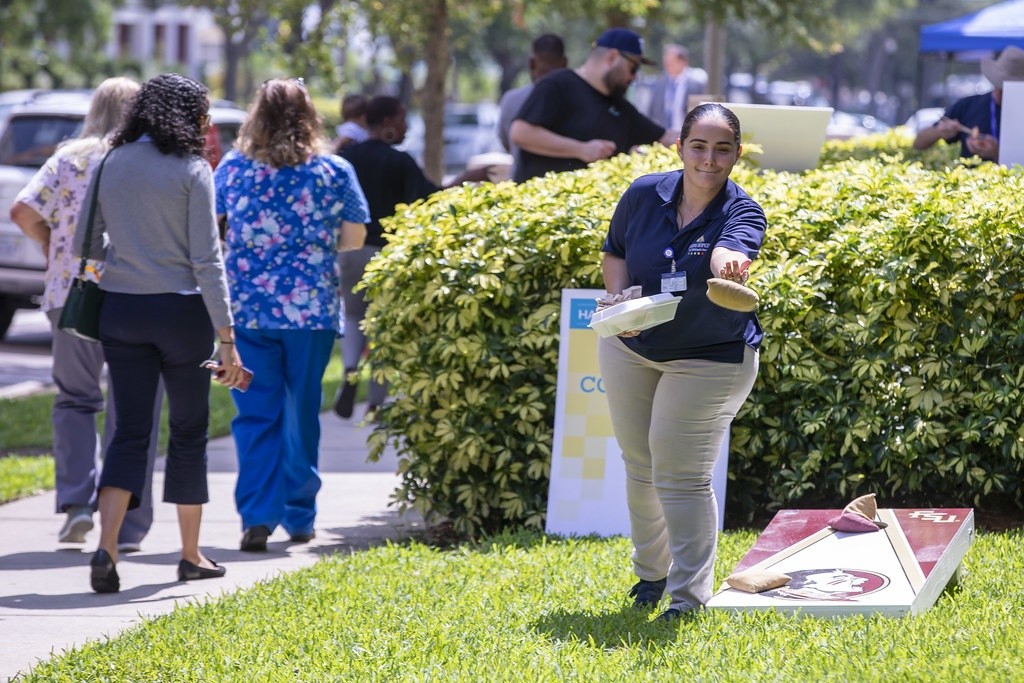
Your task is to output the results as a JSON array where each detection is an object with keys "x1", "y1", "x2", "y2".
[{"x1": 917, "y1": 1, "x2": 1024, "y2": 110}]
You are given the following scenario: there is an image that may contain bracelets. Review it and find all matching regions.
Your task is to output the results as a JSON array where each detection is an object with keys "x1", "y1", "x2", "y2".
[{"x1": 221, "y1": 341, "x2": 236, "y2": 344}]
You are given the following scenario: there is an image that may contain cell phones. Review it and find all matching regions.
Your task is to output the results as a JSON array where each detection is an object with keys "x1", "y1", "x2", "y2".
[{"x1": 215, "y1": 358, "x2": 254, "y2": 393}]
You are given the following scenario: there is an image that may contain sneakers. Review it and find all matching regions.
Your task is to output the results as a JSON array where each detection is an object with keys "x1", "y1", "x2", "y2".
[
  {"x1": 58, "y1": 507, "x2": 94, "y2": 543},
  {"x1": 627, "y1": 577, "x2": 667, "y2": 608},
  {"x1": 651, "y1": 608, "x2": 687, "y2": 622},
  {"x1": 118, "y1": 542, "x2": 140, "y2": 552}
]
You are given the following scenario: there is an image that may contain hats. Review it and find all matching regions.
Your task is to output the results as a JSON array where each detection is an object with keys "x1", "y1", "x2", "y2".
[
  {"x1": 980, "y1": 45, "x2": 1024, "y2": 89},
  {"x1": 592, "y1": 28, "x2": 658, "y2": 67}
]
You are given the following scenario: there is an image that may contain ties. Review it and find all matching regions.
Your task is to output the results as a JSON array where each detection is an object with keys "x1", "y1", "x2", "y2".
[{"x1": 663, "y1": 79, "x2": 677, "y2": 128}]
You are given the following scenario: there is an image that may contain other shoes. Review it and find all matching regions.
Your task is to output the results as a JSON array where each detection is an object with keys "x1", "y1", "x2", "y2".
[
  {"x1": 334, "y1": 368, "x2": 357, "y2": 418},
  {"x1": 291, "y1": 529, "x2": 316, "y2": 543},
  {"x1": 241, "y1": 525, "x2": 268, "y2": 551},
  {"x1": 363, "y1": 405, "x2": 377, "y2": 416}
]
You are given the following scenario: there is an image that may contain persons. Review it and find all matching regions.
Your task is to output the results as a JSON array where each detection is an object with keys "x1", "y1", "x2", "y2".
[
  {"x1": 212, "y1": 78, "x2": 372, "y2": 552},
  {"x1": 913, "y1": 44, "x2": 1024, "y2": 170},
  {"x1": 596, "y1": 103, "x2": 767, "y2": 626},
  {"x1": 510, "y1": 27, "x2": 681, "y2": 185},
  {"x1": 496, "y1": 34, "x2": 569, "y2": 179},
  {"x1": 9, "y1": 77, "x2": 164, "y2": 553},
  {"x1": 71, "y1": 73, "x2": 242, "y2": 595},
  {"x1": 648, "y1": 42, "x2": 709, "y2": 132},
  {"x1": 331, "y1": 94, "x2": 498, "y2": 425}
]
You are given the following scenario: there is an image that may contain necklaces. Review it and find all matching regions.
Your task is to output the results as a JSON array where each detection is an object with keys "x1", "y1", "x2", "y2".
[{"x1": 682, "y1": 196, "x2": 695, "y2": 220}]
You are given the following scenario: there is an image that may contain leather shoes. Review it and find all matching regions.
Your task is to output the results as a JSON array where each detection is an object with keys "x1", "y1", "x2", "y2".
[
  {"x1": 178, "y1": 559, "x2": 226, "y2": 581},
  {"x1": 90, "y1": 549, "x2": 120, "y2": 592}
]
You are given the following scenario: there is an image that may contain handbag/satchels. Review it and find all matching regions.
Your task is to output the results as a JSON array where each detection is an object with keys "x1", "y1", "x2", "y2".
[{"x1": 57, "y1": 278, "x2": 102, "y2": 344}]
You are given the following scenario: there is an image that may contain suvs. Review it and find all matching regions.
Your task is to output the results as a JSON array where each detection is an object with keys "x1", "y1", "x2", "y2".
[
  {"x1": 410, "y1": 102, "x2": 502, "y2": 173},
  {"x1": 0, "y1": 87, "x2": 251, "y2": 338}
]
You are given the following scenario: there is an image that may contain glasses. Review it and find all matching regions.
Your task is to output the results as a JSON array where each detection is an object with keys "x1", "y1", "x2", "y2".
[
  {"x1": 262, "y1": 77, "x2": 304, "y2": 86},
  {"x1": 620, "y1": 52, "x2": 640, "y2": 75}
]
